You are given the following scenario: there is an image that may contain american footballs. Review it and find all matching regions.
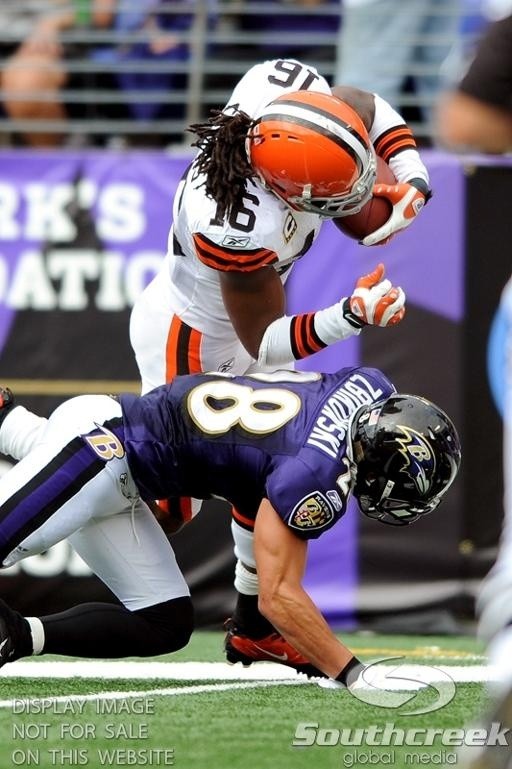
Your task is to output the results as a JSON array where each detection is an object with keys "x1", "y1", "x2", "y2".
[{"x1": 333, "y1": 152, "x2": 397, "y2": 241}]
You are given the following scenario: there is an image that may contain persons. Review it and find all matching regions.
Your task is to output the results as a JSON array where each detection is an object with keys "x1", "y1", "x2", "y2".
[
  {"x1": 444, "y1": 13, "x2": 512, "y2": 680},
  {"x1": 0, "y1": 55, "x2": 431, "y2": 679},
  {"x1": 0, "y1": 2, "x2": 512, "y2": 169},
  {"x1": 0, "y1": 368, "x2": 460, "y2": 689}
]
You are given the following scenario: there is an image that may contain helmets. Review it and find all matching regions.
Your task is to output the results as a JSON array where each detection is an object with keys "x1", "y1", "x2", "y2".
[
  {"x1": 242, "y1": 89, "x2": 376, "y2": 217},
  {"x1": 346, "y1": 393, "x2": 463, "y2": 529}
]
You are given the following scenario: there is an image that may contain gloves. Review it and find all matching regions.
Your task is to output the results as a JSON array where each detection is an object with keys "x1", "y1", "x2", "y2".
[
  {"x1": 360, "y1": 180, "x2": 432, "y2": 246},
  {"x1": 345, "y1": 264, "x2": 407, "y2": 328}
]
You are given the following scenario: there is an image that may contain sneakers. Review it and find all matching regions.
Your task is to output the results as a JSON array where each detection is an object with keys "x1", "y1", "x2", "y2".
[{"x1": 221, "y1": 615, "x2": 330, "y2": 680}]
